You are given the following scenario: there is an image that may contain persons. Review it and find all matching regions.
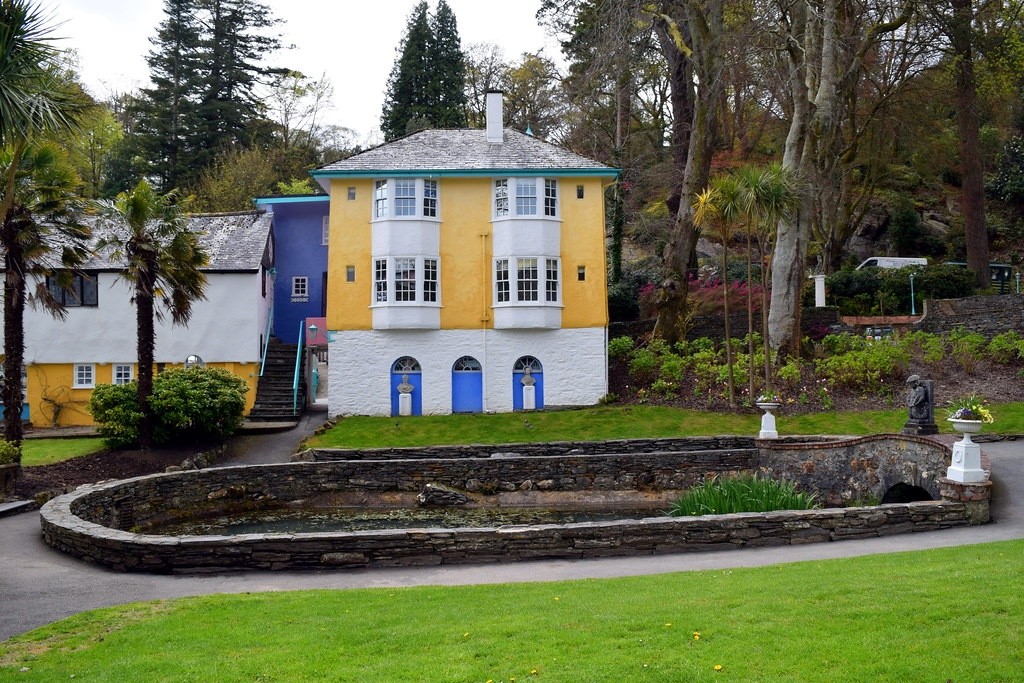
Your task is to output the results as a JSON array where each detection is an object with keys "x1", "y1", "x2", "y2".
[
  {"x1": 992, "y1": 253, "x2": 1013, "y2": 282},
  {"x1": 521, "y1": 368, "x2": 536, "y2": 386},
  {"x1": 906, "y1": 375, "x2": 928, "y2": 419},
  {"x1": 397, "y1": 374, "x2": 414, "y2": 393}
]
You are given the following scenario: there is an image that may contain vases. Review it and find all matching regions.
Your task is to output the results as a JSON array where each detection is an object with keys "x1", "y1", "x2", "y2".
[
  {"x1": 756, "y1": 403, "x2": 780, "y2": 416},
  {"x1": 947, "y1": 418, "x2": 982, "y2": 445}
]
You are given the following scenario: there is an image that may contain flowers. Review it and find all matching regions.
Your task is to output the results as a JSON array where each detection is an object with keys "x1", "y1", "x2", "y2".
[
  {"x1": 945, "y1": 391, "x2": 994, "y2": 425},
  {"x1": 753, "y1": 387, "x2": 783, "y2": 406}
]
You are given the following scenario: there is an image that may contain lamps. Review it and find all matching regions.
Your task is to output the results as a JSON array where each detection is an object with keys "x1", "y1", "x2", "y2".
[
  {"x1": 308, "y1": 320, "x2": 328, "y2": 341},
  {"x1": 1015, "y1": 270, "x2": 1021, "y2": 293},
  {"x1": 268, "y1": 267, "x2": 278, "y2": 281}
]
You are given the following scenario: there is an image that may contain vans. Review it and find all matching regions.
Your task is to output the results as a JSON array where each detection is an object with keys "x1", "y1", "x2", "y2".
[
  {"x1": 943, "y1": 260, "x2": 1012, "y2": 290},
  {"x1": 856, "y1": 257, "x2": 927, "y2": 271}
]
